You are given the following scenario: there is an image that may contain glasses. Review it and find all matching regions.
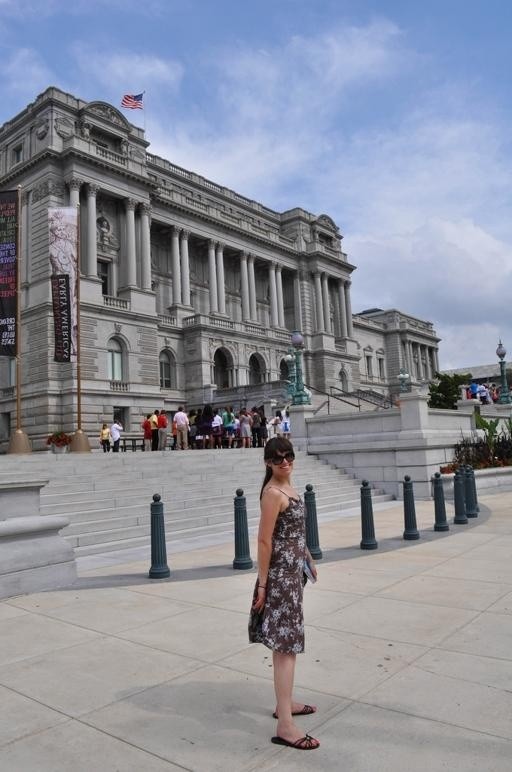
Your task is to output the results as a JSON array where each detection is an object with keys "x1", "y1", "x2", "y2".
[{"x1": 272, "y1": 452, "x2": 295, "y2": 465}]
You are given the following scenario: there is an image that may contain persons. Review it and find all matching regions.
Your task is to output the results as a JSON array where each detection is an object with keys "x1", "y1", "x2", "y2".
[
  {"x1": 470, "y1": 380, "x2": 477, "y2": 399},
  {"x1": 253, "y1": 435, "x2": 321, "y2": 751},
  {"x1": 140, "y1": 403, "x2": 291, "y2": 451},
  {"x1": 111, "y1": 420, "x2": 123, "y2": 452},
  {"x1": 491, "y1": 384, "x2": 499, "y2": 404},
  {"x1": 50, "y1": 221, "x2": 78, "y2": 355},
  {"x1": 477, "y1": 382, "x2": 488, "y2": 405},
  {"x1": 99, "y1": 423, "x2": 112, "y2": 452}
]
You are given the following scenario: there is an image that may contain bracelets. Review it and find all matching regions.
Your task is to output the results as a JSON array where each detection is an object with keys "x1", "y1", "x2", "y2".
[{"x1": 259, "y1": 585, "x2": 266, "y2": 588}]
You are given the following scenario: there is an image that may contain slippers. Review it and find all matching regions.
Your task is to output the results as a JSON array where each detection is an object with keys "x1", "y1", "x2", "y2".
[
  {"x1": 272, "y1": 734, "x2": 319, "y2": 749},
  {"x1": 273, "y1": 705, "x2": 314, "y2": 718}
]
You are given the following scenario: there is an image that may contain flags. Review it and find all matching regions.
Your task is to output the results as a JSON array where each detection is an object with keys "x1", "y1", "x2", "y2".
[{"x1": 120, "y1": 91, "x2": 144, "y2": 109}]
